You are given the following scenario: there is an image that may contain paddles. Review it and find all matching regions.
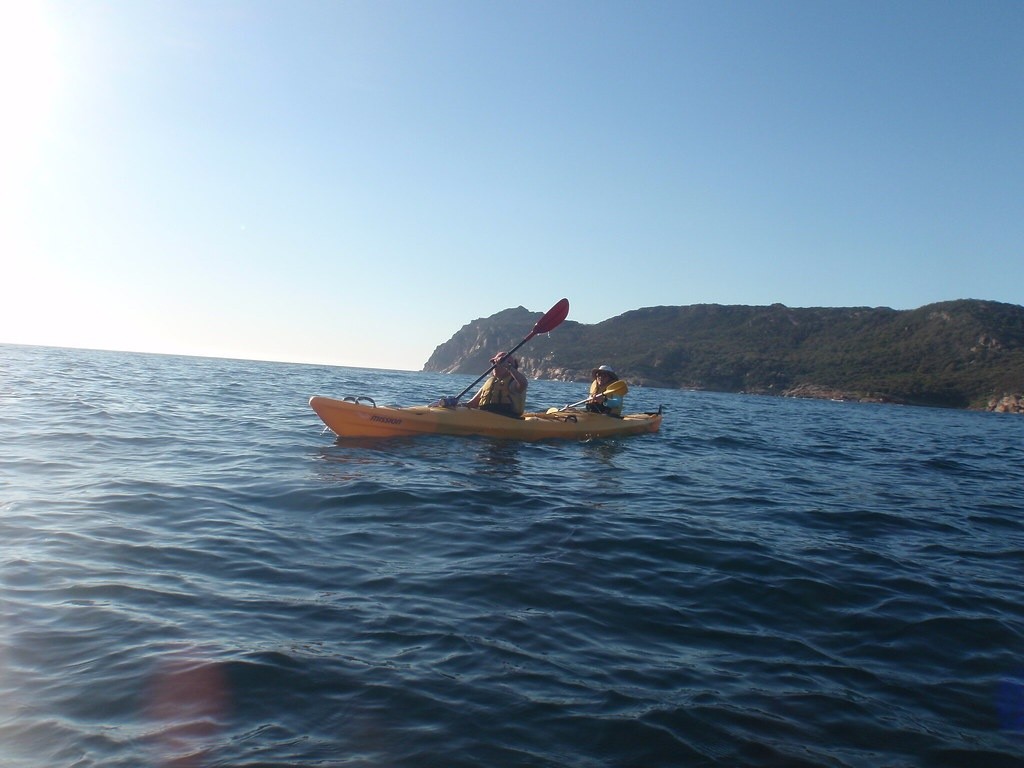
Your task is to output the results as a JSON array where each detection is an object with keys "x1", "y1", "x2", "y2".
[
  {"x1": 454, "y1": 297, "x2": 569, "y2": 399},
  {"x1": 546, "y1": 380, "x2": 628, "y2": 414}
]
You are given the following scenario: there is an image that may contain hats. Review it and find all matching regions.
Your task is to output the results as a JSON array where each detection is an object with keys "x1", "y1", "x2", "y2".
[
  {"x1": 592, "y1": 365, "x2": 618, "y2": 380},
  {"x1": 490, "y1": 352, "x2": 513, "y2": 365}
]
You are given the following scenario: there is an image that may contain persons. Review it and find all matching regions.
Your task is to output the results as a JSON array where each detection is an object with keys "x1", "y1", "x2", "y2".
[
  {"x1": 566, "y1": 365, "x2": 623, "y2": 416},
  {"x1": 466, "y1": 351, "x2": 528, "y2": 420}
]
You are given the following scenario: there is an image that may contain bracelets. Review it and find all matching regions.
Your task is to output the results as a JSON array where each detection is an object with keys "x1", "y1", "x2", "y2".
[{"x1": 508, "y1": 363, "x2": 511, "y2": 371}]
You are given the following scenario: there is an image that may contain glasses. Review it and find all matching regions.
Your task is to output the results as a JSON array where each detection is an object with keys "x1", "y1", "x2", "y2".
[{"x1": 596, "y1": 372, "x2": 610, "y2": 377}]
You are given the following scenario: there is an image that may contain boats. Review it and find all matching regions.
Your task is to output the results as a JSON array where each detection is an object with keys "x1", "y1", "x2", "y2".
[{"x1": 311, "y1": 396, "x2": 665, "y2": 443}]
